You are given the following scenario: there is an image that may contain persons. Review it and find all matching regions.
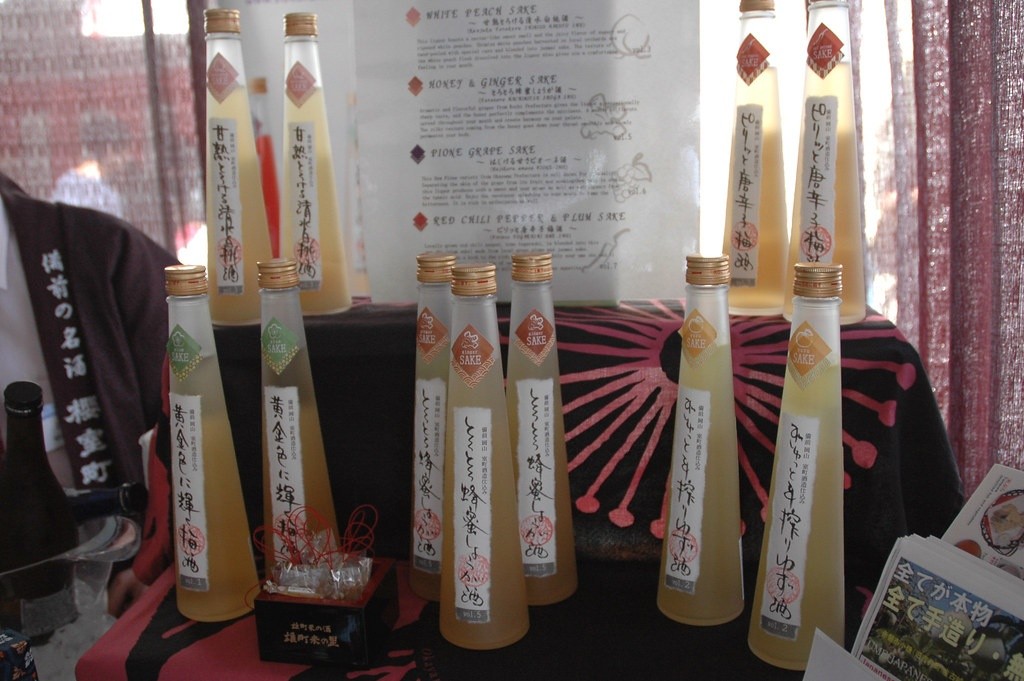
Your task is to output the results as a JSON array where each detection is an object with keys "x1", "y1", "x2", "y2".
[
  {"x1": 51, "y1": 109, "x2": 133, "y2": 219},
  {"x1": 0, "y1": 173, "x2": 183, "y2": 615}
]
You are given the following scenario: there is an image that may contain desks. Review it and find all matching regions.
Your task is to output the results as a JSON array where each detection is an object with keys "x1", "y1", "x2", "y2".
[
  {"x1": 73, "y1": 569, "x2": 884, "y2": 681},
  {"x1": 150, "y1": 306, "x2": 964, "y2": 580}
]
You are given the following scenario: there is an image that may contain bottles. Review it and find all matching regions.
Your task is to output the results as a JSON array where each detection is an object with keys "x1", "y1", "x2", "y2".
[
  {"x1": 165, "y1": 266, "x2": 260, "y2": 624},
  {"x1": 749, "y1": 263, "x2": 845, "y2": 669},
  {"x1": 439, "y1": 264, "x2": 529, "y2": 648},
  {"x1": 411, "y1": 254, "x2": 455, "y2": 600},
  {"x1": 255, "y1": 259, "x2": 344, "y2": 587},
  {"x1": 507, "y1": 253, "x2": 579, "y2": 608},
  {"x1": 657, "y1": 255, "x2": 744, "y2": 626},
  {"x1": 277, "y1": 12, "x2": 353, "y2": 318},
  {"x1": 781, "y1": 1, "x2": 868, "y2": 323},
  {"x1": 206, "y1": 10, "x2": 275, "y2": 322},
  {"x1": 720, "y1": 1, "x2": 788, "y2": 317}
]
